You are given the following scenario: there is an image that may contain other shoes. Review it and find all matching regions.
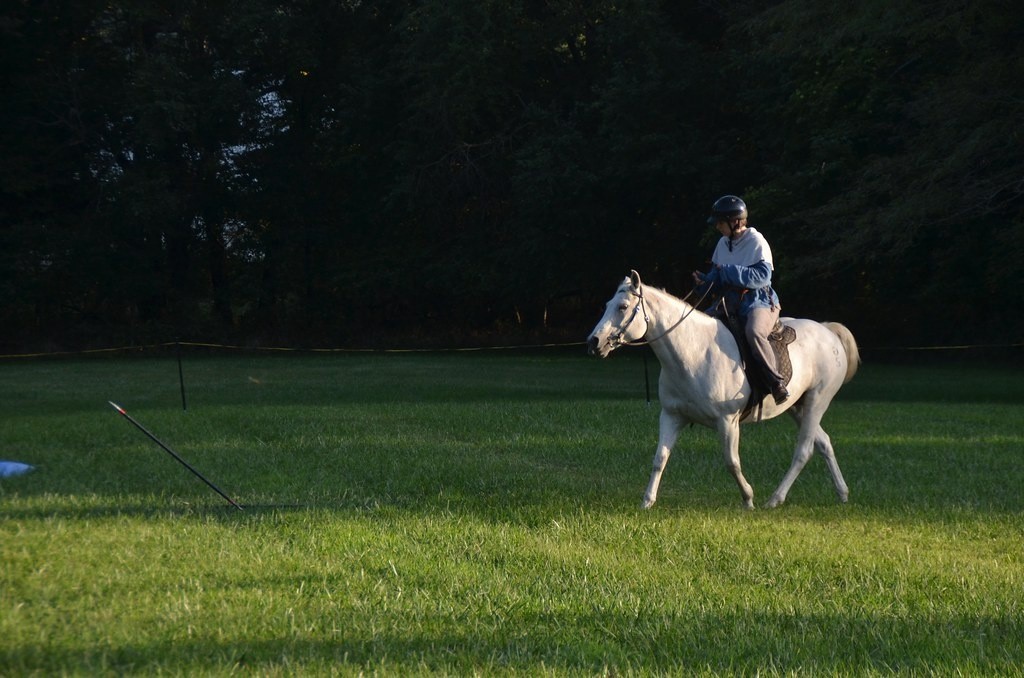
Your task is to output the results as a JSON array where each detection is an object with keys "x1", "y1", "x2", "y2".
[{"x1": 771, "y1": 379, "x2": 788, "y2": 403}]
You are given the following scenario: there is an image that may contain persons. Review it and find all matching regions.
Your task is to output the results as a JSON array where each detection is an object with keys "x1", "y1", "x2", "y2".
[{"x1": 692, "y1": 193, "x2": 790, "y2": 407}]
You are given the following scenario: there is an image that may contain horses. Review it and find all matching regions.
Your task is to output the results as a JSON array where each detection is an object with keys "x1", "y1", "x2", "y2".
[{"x1": 588, "y1": 270, "x2": 859, "y2": 511}]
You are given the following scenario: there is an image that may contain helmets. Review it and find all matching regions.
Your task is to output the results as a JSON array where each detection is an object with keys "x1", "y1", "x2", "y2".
[{"x1": 707, "y1": 195, "x2": 748, "y2": 233}]
